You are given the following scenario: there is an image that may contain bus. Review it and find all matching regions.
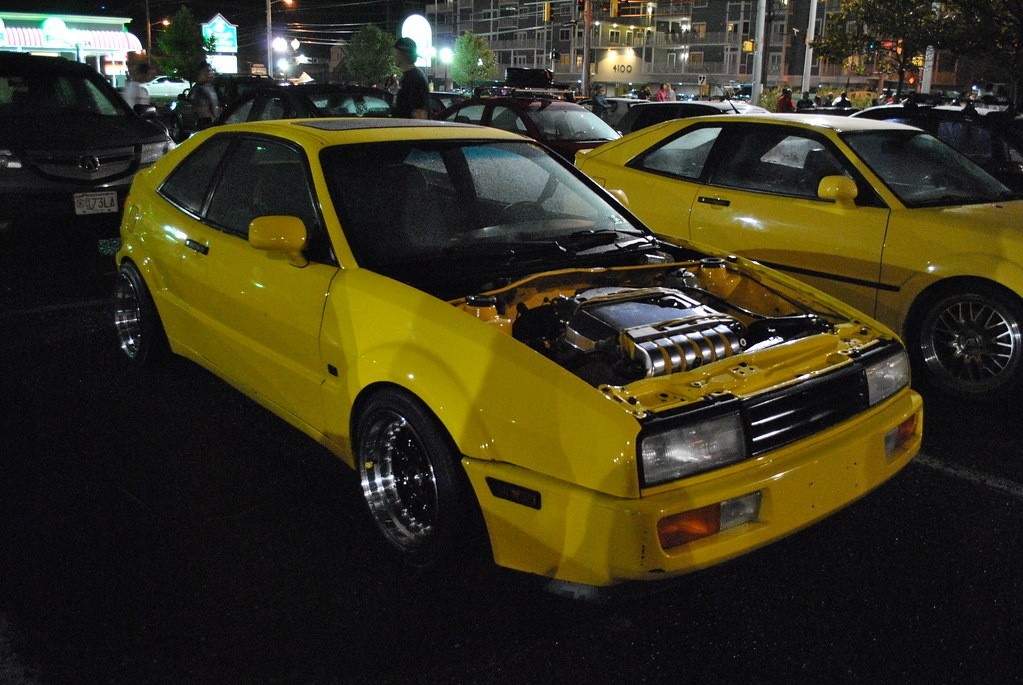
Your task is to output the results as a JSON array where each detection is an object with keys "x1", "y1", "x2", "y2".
[
  {"x1": 722, "y1": 82, "x2": 752, "y2": 99},
  {"x1": 505, "y1": 68, "x2": 555, "y2": 88}
]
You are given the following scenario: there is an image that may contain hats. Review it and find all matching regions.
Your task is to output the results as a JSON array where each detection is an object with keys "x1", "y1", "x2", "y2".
[
  {"x1": 193, "y1": 61, "x2": 210, "y2": 71},
  {"x1": 392, "y1": 38, "x2": 416, "y2": 51}
]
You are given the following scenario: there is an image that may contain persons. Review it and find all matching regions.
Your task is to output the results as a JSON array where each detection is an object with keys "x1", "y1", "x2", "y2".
[
  {"x1": 592, "y1": 84, "x2": 611, "y2": 118},
  {"x1": 657, "y1": 82, "x2": 676, "y2": 102},
  {"x1": 833, "y1": 90, "x2": 852, "y2": 107},
  {"x1": 797, "y1": 91, "x2": 814, "y2": 109},
  {"x1": 777, "y1": 88, "x2": 796, "y2": 113},
  {"x1": 122, "y1": 56, "x2": 157, "y2": 109},
  {"x1": 189, "y1": 61, "x2": 219, "y2": 129},
  {"x1": 389, "y1": 37, "x2": 429, "y2": 119},
  {"x1": 638, "y1": 84, "x2": 653, "y2": 100},
  {"x1": 879, "y1": 93, "x2": 893, "y2": 105},
  {"x1": 822, "y1": 92, "x2": 833, "y2": 107},
  {"x1": 811, "y1": 95, "x2": 822, "y2": 107}
]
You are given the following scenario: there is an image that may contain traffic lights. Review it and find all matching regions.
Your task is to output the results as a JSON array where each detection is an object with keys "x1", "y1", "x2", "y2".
[
  {"x1": 867, "y1": 44, "x2": 874, "y2": 50},
  {"x1": 545, "y1": 2, "x2": 554, "y2": 22},
  {"x1": 908, "y1": 67, "x2": 919, "y2": 88},
  {"x1": 578, "y1": 0, "x2": 584, "y2": 11},
  {"x1": 610, "y1": 0, "x2": 622, "y2": 18}
]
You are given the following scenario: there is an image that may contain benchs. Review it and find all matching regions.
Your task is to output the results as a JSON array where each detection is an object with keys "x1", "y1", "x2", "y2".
[{"x1": 232, "y1": 163, "x2": 379, "y2": 224}]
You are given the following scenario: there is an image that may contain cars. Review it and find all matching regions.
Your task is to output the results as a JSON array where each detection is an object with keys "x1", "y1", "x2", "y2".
[
  {"x1": 114, "y1": 119, "x2": 924, "y2": 587},
  {"x1": 0, "y1": 50, "x2": 179, "y2": 261},
  {"x1": 133, "y1": 74, "x2": 1021, "y2": 193},
  {"x1": 574, "y1": 114, "x2": 1022, "y2": 412}
]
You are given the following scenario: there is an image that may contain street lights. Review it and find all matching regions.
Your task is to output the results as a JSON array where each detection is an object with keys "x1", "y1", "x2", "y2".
[
  {"x1": 439, "y1": 46, "x2": 453, "y2": 92},
  {"x1": 266, "y1": 0, "x2": 292, "y2": 79},
  {"x1": 147, "y1": 20, "x2": 170, "y2": 81}
]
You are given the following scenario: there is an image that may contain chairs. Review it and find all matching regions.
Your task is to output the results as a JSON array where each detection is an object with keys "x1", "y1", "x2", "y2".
[
  {"x1": 377, "y1": 163, "x2": 453, "y2": 250},
  {"x1": 804, "y1": 142, "x2": 854, "y2": 194}
]
